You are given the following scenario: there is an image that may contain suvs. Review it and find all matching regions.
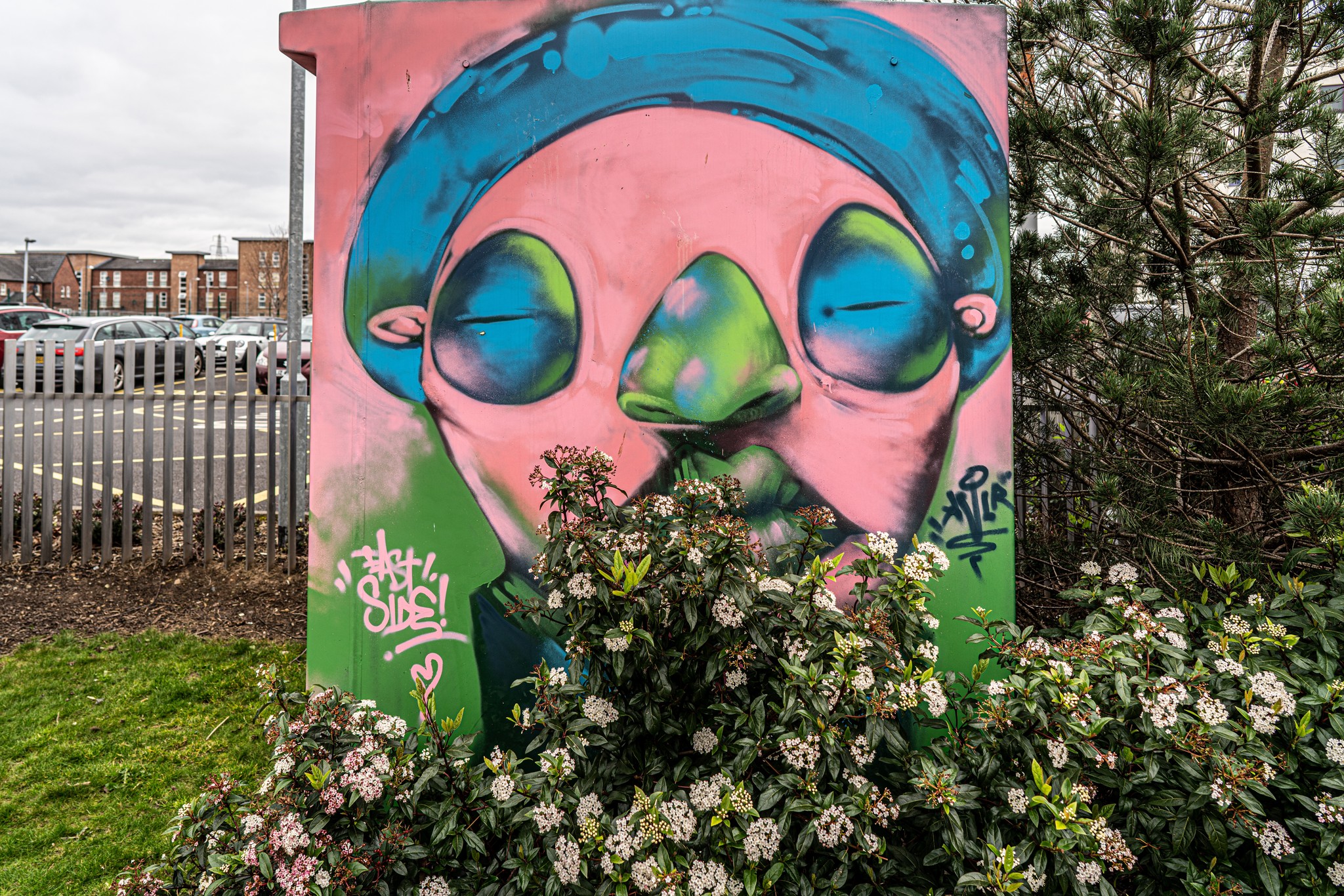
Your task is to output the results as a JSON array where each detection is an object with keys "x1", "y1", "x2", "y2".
[
  {"x1": 0, "y1": 302, "x2": 232, "y2": 392},
  {"x1": 193, "y1": 316, "x2": 287, "y2": 373},
  {"x1": 256, "y1": 315, "x2": 313, "y2": 396}
]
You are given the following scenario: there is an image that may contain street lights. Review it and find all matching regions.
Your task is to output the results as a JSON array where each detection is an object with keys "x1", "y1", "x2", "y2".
[
  {"x1": 244, "y1": 281, "x2": 249, "y2": 316},
  {"x1": 190, "y1": 277, "x2": 201, "y2": 311},
  {"x1": 203, "y1": 271, "x2": 209, "y2": 316},
  {"x1": 22, "y1": 238, "x2": 36, "y2": 326},
  {"x1": 80, "y1": 265, "x2": 93, "y2": 316}
]
